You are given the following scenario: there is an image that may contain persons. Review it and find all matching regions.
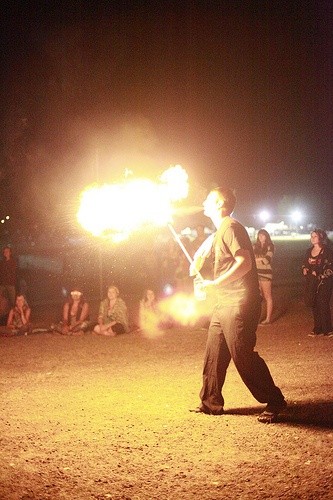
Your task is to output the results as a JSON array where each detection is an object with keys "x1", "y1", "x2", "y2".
[
  {"x1": 301, "y1": 229, "x2": 333, "y2": 337},
  {"x1": 252, "y1": 230, "x2": 274, "y2": 326},
  {"x1": 0, "y1": 224, "x2": 214, "y2": 343},
  {"x1": 187, "y1": 186, "x2": 286, "y2": 423}
]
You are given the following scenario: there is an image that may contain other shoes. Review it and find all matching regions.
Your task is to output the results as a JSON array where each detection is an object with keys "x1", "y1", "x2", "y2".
[
  {"x1": 308, "y1": 330, "x2": 324, "y2": 337},
  {"x1": 326, "y1": 331, "x2": 333, "y2": 337}
]
